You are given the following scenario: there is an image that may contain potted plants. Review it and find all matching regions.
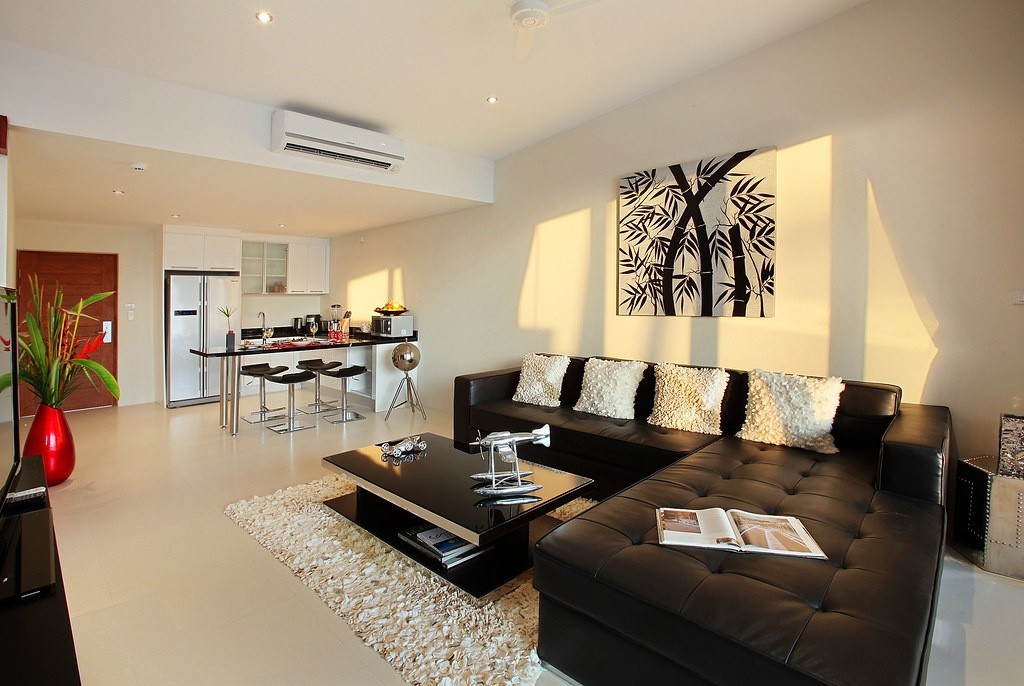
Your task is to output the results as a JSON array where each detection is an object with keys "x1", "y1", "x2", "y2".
[{"x1": 215, "y1": 304, "x2": 238, "y2": 350}]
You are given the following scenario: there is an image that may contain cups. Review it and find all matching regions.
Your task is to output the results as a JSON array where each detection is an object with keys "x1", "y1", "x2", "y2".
[{"x1": 293, "y1": 318, "x2": 303, "y2": 329}]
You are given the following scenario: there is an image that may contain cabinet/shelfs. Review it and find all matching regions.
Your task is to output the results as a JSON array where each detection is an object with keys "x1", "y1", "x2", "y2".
[
  {"x1": 237, "y1": 336, "x2": 419, "y2": 413},
  {"x1": 0, "y1": 454, "x2": 81, "y2": 686},
  {"x1": 163, "y1": 225, "x2": 330, "y2": 295}
]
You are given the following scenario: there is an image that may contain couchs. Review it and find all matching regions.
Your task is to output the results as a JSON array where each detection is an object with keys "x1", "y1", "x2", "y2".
[{"x1": 453, "y1": 355, "x2": 951, "y2": 686}]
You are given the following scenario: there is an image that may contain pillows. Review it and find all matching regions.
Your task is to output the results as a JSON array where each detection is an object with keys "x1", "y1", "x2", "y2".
[
  {"x1": 735, "y1": 367, "x2": 845, "y2": 455},
  {"x1": 511, "y1": 353, "x2": 571, "y2": 407},
  {"x1": 573, "y1": 357, "x2": 648, "y2": 419},
  {"x1": 645, "y1": 360, "x2": 730, "y2": 436}
]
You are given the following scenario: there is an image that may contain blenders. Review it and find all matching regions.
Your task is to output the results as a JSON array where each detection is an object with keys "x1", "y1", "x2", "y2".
[{"x1": 328, "y1": 304, "x2": 342, "y2": 333}]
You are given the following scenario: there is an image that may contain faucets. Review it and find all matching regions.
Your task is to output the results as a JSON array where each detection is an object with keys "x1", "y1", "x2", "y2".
[{"x1": 258, "y1": 311, "x2": 265, "y2": 332}]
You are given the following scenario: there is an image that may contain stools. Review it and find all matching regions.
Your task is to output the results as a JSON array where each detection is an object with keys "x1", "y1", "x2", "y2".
[{"x1": 240, "y1": 358, "x2": 368, "y2": 435}]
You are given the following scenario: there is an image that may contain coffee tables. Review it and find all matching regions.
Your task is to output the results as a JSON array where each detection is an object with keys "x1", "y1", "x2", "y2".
[{"x1": 321, "y1": 432, "x2": 595, "y2": 609}]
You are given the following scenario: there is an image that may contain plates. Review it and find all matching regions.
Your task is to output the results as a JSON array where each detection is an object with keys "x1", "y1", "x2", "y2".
[
  {"x1": 290, "y1": 342, "x2": 311, "y2": 346},
  {"x1": 375, "y1": 310, "x2": 411, "y2": 316},
  {"x1": 238, "y1": 344, "x2": 260, "y2": 346}
]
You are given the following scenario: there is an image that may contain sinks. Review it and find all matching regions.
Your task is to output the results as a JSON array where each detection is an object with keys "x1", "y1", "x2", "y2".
[{"x1": 241, "y1": 334, "x2": 262, "y2": 337}]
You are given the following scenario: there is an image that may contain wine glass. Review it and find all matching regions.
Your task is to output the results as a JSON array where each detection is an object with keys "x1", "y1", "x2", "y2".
[
  {"x1": 265, "y1": 327, "x2": 274, "y2": 344},
  {"x1": 310, "y1": 321, "x2": 318, "y2": 341}
]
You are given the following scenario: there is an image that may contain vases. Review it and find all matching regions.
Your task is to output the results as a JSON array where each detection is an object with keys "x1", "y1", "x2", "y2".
[{"x1": 21, "y1": 402, "x2": 76, "y2": 488}]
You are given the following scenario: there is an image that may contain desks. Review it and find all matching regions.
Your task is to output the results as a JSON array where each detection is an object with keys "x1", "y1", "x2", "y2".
[{"x1": 190, "y1": 335, "x2": 377, "y2": 437}]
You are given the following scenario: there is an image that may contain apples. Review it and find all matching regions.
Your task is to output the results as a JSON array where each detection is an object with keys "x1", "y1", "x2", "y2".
[{"x1": 375, "y1": 301, "x2": 405, "y2": 310}]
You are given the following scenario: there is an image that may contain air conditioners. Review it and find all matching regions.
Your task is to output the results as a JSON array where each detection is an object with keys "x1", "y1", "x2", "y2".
[{"x1": 270, "y1": 109, "x2": 406, "y2": 175}]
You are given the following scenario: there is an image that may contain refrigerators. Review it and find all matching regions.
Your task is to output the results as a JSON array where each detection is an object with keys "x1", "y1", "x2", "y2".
[{"x1": 164, "y1": 274, "x2": 244, "y2": 409}]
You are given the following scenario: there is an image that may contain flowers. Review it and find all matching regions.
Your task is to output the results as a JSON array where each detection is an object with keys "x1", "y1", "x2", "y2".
[{"x1": 0, "y1": 272, "x2": 120, "y2": 411}]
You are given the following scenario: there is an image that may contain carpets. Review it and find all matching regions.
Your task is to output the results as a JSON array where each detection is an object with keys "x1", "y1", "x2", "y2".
[{"x1": 224, "y1": 471, "x2": 600, "y2": 686}]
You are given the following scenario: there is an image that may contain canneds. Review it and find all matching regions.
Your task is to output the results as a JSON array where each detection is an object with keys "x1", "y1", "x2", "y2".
[{"x1": 328, "y1": 331, "x2": 343, "y2": 343}]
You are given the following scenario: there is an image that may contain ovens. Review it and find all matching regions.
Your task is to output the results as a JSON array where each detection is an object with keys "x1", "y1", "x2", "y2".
[{"x1": 371, "y1": 315, "x2": 414, "y2": 338}]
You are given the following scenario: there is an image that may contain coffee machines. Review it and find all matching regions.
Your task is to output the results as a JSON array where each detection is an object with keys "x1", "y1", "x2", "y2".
[{"x1": 306, "y1": 314, "x2": 320, "y2": 334}]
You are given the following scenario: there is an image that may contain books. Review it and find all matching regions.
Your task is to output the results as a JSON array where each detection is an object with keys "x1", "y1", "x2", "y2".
[
  {"x1": 398, "y1": 527, "x2": 494, "y2": 569},
  {"x1": 655, "y1": 508, "x2": 827, "y2": 561}
]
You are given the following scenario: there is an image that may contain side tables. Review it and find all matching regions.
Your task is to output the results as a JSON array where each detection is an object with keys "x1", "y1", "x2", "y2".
[{"x1": 951, "y1": 454, "x2": 1024, "y2": 581}]
